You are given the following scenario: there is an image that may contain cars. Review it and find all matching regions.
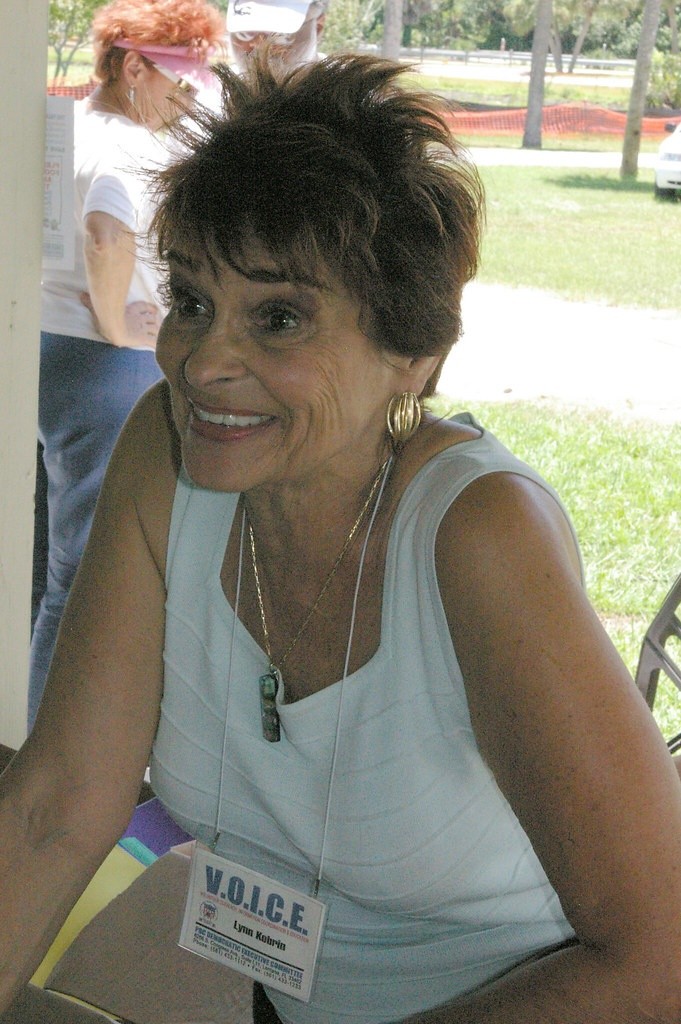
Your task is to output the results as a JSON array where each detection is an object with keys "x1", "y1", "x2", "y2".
[{"x1": 653, "y1": 121, "x2": 681, "y2": 198}]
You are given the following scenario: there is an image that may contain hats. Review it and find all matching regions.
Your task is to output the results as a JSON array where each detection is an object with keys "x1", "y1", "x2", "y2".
[
  {"x1": 112, "y1": 38, "x2": 221, "y2": 96},
  {"x1": 224, "y1": 0, "x2": 318, "y2": 34}
]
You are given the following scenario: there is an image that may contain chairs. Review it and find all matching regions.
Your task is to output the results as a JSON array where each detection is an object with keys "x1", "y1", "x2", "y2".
[{"x1": 636, "y1": 574, "x2": 681, "y2": 756}]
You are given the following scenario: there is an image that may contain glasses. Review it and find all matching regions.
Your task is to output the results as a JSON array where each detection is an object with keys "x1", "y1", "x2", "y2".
[
  {"x1": 143, "y1": 56, "x2": 195, "y2": 101},
  {"x1": 234, "y1": 13, "x2": 327, "y2": 46}
]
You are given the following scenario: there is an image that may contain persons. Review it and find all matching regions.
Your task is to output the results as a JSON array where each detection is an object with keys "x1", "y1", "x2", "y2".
[
  {"x1": 0, "y1": 50, "x2": 681, "y2": 1024},
  {"x1": 28, "y1": 0, "x2": 230, "y2": 752},
  {"x1": 226, "y1": 0, "x2": 334, "y2": 101}
]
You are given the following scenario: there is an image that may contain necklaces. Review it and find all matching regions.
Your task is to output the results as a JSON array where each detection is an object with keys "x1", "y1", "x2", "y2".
[{"x1": 242, "y1": 424, "x2": 398, "y2": 742}]
